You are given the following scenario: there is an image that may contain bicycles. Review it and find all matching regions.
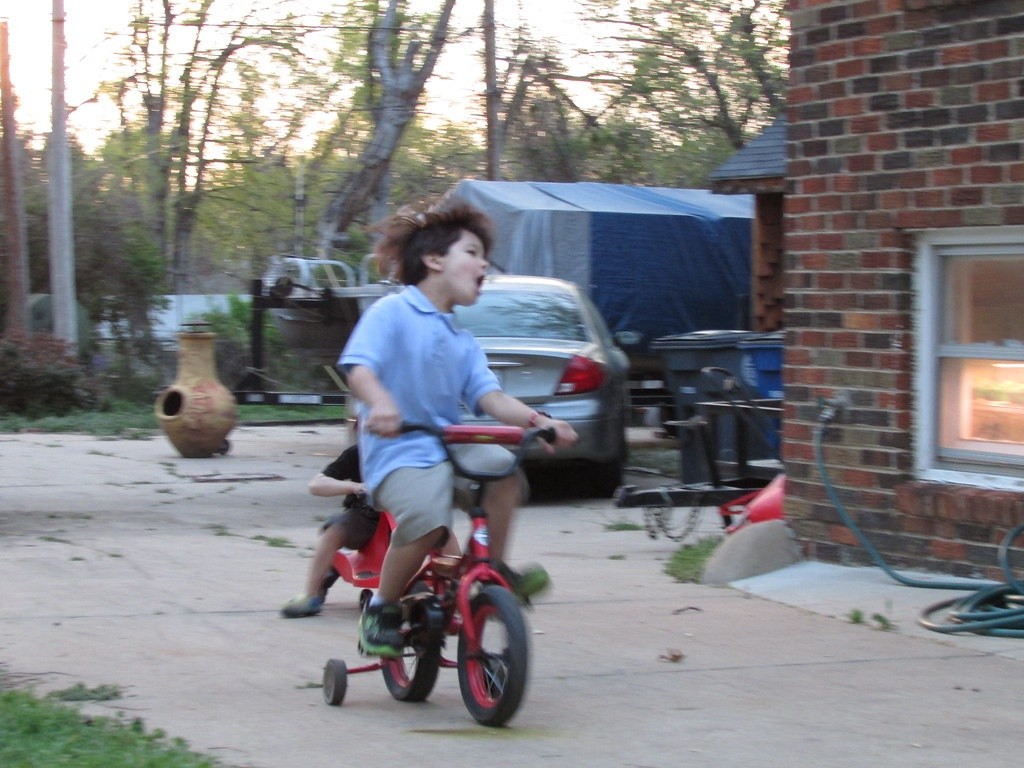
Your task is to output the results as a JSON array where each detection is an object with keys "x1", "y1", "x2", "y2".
[{"x1": 319, "y1": 418, "x2": 558, "y2": 729}]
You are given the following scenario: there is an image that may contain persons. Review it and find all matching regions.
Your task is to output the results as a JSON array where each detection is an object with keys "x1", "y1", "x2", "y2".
[
  {"x1": 334, "y1": 198, "x2": 579, "y2": 658},
  {"x1": 280, "y1": 416, "x2": 385, "y2": 618}
]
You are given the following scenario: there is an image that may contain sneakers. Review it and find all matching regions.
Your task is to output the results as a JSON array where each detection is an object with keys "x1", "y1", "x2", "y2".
[
  {"x1": 357, "y1": 598, "x2": 404, "y2": 657},
  {"x1": 496, "y1": 566, "x2": 548, "y2": 597}
]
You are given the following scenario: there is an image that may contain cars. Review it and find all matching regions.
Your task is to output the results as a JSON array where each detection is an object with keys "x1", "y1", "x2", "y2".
[{"x1": 451, "y1": 273, "x2": 644, "y2": 500}]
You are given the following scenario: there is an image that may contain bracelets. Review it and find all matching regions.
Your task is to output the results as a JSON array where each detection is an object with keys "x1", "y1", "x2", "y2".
[{"x1": 524, "y1": 411, "x2": 556, "y2": 428}]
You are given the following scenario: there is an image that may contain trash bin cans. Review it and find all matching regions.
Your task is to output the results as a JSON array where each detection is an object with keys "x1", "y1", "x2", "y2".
[
  {"x1": 735, "y1": 330, "x2": 785, "y2": 478},
  {"x1": 651, "y1": 327, "x2": 754, "y2": 487}
]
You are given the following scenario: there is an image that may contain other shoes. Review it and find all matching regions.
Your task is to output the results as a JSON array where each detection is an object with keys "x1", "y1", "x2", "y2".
[{"x1": 279, "y1": 594, "x2": 321, "y2": 617}]
